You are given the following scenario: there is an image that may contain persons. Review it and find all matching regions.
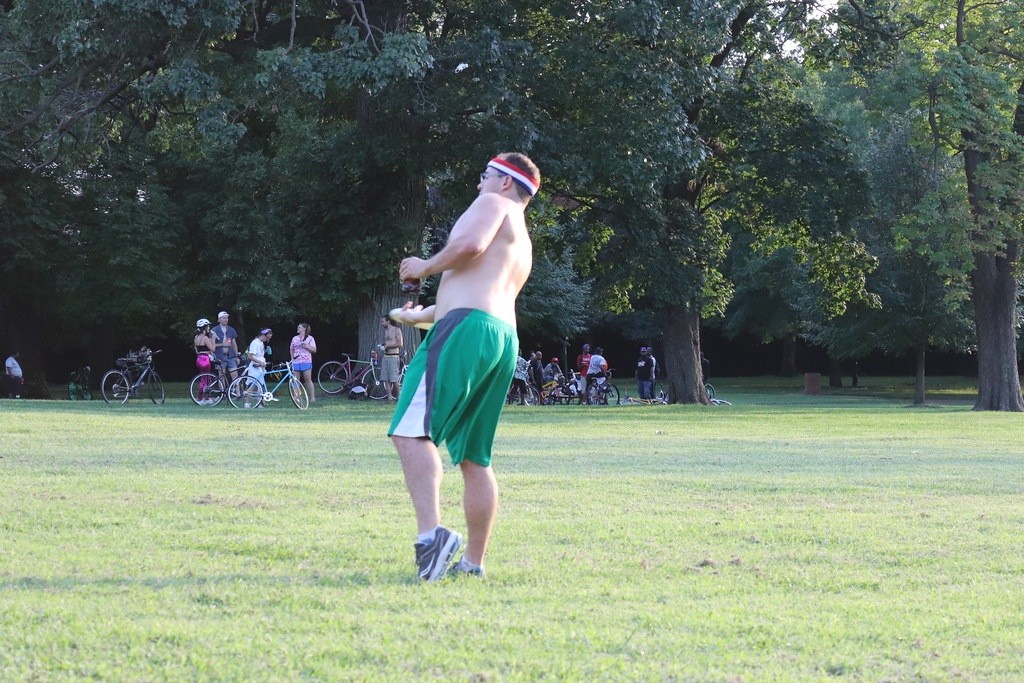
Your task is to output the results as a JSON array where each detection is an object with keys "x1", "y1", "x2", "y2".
[
  {"x1": 852, "y1": 361, "x2": 859, "y2": 386},
  {"x1": 244, "y1": 328, "x2": 273, "y2": 409},
  {"x1": 194, "y1": 319, "x2": 216, "y2": 401},
  {"x1": 506, "y1": 343, "x2": 661, "y2": 405},
  {"x1": 290, "y1": 323, "x2": 316, "y2": 402},
  {"x1": 378, "y1": 314, "x2": 403, "y2": 402},
  {"x1": 700, "y1": 352, "x2": 710, "y2": 385},
  {"x1": 387, "y1": 152, "x2": 540, "y2": 582},
  {"x1": 5, "y1": 349, "x2": 23, "y2": 399},
  {"x1": 209, "y1": 311, "x2": 241, "y2": 400}
]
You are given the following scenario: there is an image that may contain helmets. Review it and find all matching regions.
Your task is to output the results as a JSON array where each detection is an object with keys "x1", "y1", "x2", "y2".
[
  {"x1": 349, "y1": 386, "x2": 368, "y2": 399},
  {"x1": 196, "y1": 318, "x2": 212, "y2": 328}
]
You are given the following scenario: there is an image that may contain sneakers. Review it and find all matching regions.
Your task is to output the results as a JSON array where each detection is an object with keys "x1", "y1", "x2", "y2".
[
  {"x1": 446, "y1": 554, "x2": 486, "y2": 583},
  {"x1": 413, "y1": 525, "x2": 462, "y2": 586}
]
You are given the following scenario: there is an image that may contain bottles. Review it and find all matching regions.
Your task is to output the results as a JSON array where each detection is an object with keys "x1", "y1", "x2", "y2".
[
  {"x1": 402, "y1": 278, "x2": 421, "y2": 296},
  {"x1": 210, "y1": 330, "x2": 214, "y2": 334},
  {"x1": 227, "y1": 338, "x2": 231, "y2": 346}
]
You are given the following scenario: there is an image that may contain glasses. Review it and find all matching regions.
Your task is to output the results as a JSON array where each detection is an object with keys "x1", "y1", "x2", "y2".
[
  {"x1": 298, "y1": 326, "x2": 305, "y2": 329},
  {"x1": 265, "y1": 335, "x2": 271, "y2": 339},
  {"x1": 480, "y1": 169, "x2": 505, "y2": 183}
]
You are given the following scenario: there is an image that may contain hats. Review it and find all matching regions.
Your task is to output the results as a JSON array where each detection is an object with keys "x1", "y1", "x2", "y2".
[
  {"x1": 552, "y1": 357, "x2": 558, "y2": 362},
  {"x1": 584, "y1": 344, "x2": 589, "y2": 350},
  {"x1": 218, "y1": 311, "x2": 229, "y2": 322},
  {"x1": 640, "y1": 347, "x2": 646, "y2": 352}
]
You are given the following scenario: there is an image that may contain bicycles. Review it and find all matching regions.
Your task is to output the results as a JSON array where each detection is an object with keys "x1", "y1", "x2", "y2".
[
  {"x1": 227, "y1": 354, "x2": 309, "y2": 409},
  {"x1": 101, "y1": 347, "x2": 166, "y2": 405},
  {"x1": 317, "y1": 351, "x2": 394, "y2": 399},
  {"x1": 189, "y1": 350, "x2": 270, "y2": 408},
  {"x1": 68, "y1": 365, "x2": 93, "y2": 401},
  {"x1": 509, "y1": 368, "x2": 670, "y2": 407}
]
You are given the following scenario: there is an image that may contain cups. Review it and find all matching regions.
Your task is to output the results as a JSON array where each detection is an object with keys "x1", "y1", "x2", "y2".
[{"x1": 267, "y1": 346, "x2": 272, "y2": 355}]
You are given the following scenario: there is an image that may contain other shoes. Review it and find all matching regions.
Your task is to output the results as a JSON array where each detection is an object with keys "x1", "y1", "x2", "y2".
[
  {"x1": 244, "y1": 403, "x2": 252, "y2": 409},
  {"x1": 197, "y1": 400, "x2": 206, "y2": 405},
  {"x1": 206, "y1": 399, "x2": 215, "y2": 404},
  {"x1": 257, "y1": 404, "x2": 264, "y2": 408}
]
